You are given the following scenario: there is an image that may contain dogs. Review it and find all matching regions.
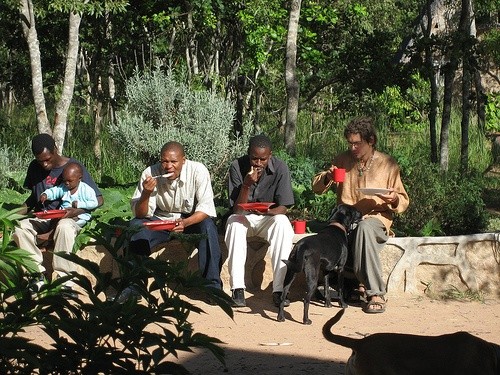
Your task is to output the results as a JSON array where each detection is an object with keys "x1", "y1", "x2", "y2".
[
  {"x1": 277, "y1": 204, "x2": 361, "y2": 325},
  {"x1": 322, "y1": 309, "x2": 500, "y2": 375}
]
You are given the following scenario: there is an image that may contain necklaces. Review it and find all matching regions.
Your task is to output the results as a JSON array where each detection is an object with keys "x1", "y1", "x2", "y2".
[{"x1": 356, "y1": 150, "x2": 375, "y2": 176}]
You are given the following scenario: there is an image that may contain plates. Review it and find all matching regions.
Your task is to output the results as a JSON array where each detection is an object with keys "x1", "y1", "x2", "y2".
[
  {"x1": 143, "y1": 222, "x2": 176, "y2": 231},
  {"x1": 237, "y1": 202, "x2": 274, "y2": 210},
  {"x1": 34, "y1": 211, "x2": 65, "y2": 219},
  {"x1": 356, "y1": 189, "x2": 393, "y2": 196}
]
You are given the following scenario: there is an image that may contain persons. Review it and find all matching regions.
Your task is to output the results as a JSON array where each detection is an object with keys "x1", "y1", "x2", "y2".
[
  {"x1": 107, "y1": 141, "x2": 228, "y2": 305},
  {"x1": 16, "y1": 133, "x2": 104, "y2": 298},
  {"x1": 225, "y1": 135, "x2": 294, "y2": 307},
  {"x1": 312, "y1": 118, "x2": 409, "y2": 314},
  {"x1": 37, "y1": 162, "x2": 97, "y2": 241}
]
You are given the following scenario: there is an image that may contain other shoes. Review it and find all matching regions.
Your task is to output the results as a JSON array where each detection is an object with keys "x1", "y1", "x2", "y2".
[
  {"x1": 106, "y1": 287, "x2": 142, "y2": 304},
  {"x1": 273, "y1": 292, "x2": 290, "y2": 307},
  {"x1": 231, "y1": 288, "x2": 246, "y2": 306}
]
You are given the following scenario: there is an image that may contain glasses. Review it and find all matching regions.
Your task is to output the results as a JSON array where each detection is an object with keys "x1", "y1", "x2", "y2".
[{"x1": 346, "y1": 142, "x2": 363, "y2": 148}]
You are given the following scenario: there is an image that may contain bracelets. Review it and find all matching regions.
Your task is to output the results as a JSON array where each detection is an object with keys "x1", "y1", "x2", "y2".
[{"x1": 243, "y1": 183, "x2": 251, "y2": 188}]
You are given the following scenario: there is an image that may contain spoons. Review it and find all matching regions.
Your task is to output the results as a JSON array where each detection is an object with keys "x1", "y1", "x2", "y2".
[{"x1": 42, "y1": 200, "x2": 48, "y2": 214}]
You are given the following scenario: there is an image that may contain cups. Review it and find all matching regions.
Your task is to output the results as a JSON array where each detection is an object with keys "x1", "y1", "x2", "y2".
[
  {"x1": 294, "y1": 221, "x2": 305, "y2": 233},
  {"x1": 333, "y1": 169, "x2": 345, "y2": 182}
]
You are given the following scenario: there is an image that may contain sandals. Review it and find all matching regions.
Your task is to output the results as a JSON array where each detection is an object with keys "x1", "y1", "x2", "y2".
[{"x1": 364, "y1": 295, "x2": 388, "y2": 313}]
